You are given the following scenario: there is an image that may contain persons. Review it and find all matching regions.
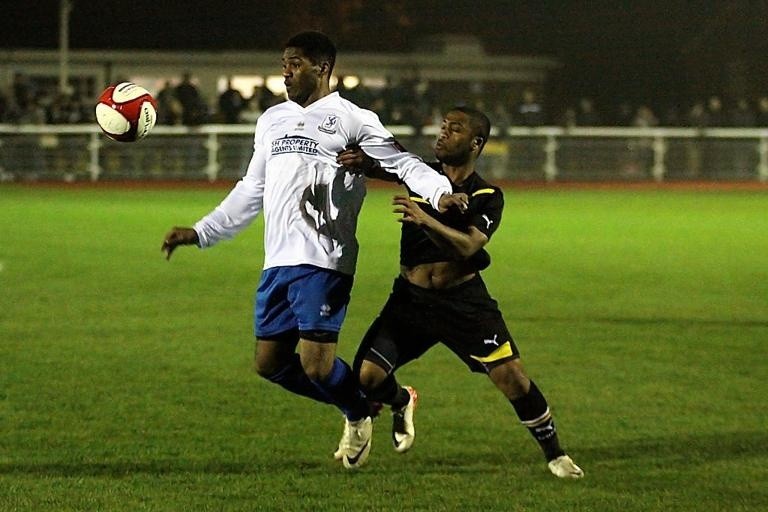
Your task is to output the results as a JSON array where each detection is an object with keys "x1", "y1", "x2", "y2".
[
  {"x1": 334, "y1": 104, "x2": 588, "y2": 484},
  {"x1": 157, "y1": 31, "x2": 473, "y2": 471},
  {"x1": 1, "y1": 59, "x2": 766, "y2": 182}
]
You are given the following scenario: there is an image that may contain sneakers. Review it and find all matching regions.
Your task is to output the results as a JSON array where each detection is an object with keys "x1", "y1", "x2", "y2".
[
  {"x1": 390, "y1": 385, "x2": 417, "y2": 454},
  {"x1": 547, "y1": 455, "x2": 584, "y2": 480},
  {"x1": 333, "y1": 414, "x2": 346, "y2": 461},
  {"x1": 342, "y1": 413, "x2": 372, "y2": 471}
]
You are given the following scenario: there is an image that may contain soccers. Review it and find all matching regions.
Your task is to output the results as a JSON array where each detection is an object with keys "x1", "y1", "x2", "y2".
[{"x1": 97, "y1": 82, "x2": 157, "y2": 142}]
